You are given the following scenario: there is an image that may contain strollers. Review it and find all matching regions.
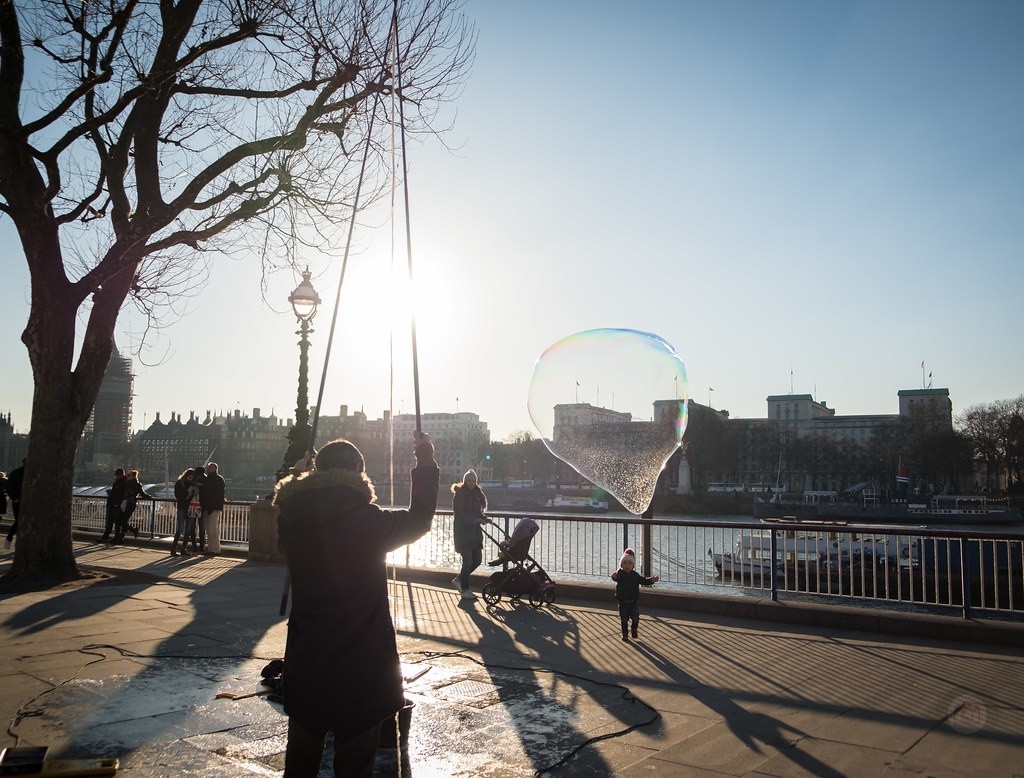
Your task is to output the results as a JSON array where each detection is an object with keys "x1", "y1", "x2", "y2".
[{"x1": 479, "y1": 518, "x2": 558, "y2": 608}]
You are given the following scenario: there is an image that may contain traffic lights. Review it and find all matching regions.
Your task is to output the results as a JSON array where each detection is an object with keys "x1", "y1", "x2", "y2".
[{"x1": 486, "y1": 454, "x2": 491, "y2": 460}]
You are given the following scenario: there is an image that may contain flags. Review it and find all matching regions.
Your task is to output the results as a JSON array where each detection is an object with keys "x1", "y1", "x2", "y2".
[{"x1": 186, "y1": 492, "x2": 201, "y2": 517}]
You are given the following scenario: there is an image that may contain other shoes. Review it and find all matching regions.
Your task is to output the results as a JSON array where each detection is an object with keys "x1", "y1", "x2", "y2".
[
  {"x1": 462, "y1": 590, "x2": 478, "y2": 599},
  {"x1": 97, "y1": 534, "x2": 108, "y2": 542},
  {"x1": 451, "y1": 577, "x2": 462, "y2": 594},
  {"x1": 206, "y1": 551, "x2": 219, "y2": 556},
  {"x1": 5, "y1": 539, "x2": 12, "y2": 550},
  {"x1": 109, "y1": 534, "x2": 120, "y2": 542},
  {"x1": 631, "y1": 629, "x2": 638, "y2": 638},
  {"x1": 621, "y1": 634, "x2": 628, "y2": 641}
]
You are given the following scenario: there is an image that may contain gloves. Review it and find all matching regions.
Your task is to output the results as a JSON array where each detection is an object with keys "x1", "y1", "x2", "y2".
[
  {"x1": 485, "y1": 518, "x2": 492, "y2": 523},
  {"x1": 474, "y1": 518, "x2": 485, "y2": 525}
]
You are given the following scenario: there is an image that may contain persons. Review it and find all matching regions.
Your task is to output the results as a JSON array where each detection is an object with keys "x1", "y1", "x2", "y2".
[
  {"x1": 110, "y1": 470, "x2": 152, "y2": 543},
  {"x1": 0, "y1": 472, "x2": 8, "y2": 529},
  {"x1": 97, "y1": 467, "x2": 125, "y2": 542},
  {"x1": 271, "y1": 429, "x2": 439, "y2": 778},
  {"x1": 612, "y1": 548, "x2": 659, "y2": 641},
  {"x1": 6, "y1": 457, "x2": 27, "y2": 550},
  {"x1": 202, "y1": 462, "x2": 225, "y2": 557},
  {"x1": 450, "y1": 468, "x2": 488, "y2": 599},
  {"x1": 171, "y1": 467, "x2": 207, "y2": 556},
  {"x1": 488, "y1": 539, "x2": 510, "y2": 564}
]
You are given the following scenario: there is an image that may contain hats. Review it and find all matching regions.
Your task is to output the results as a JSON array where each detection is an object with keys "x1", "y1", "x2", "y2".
[
  {"x1": 463, "y1": 469, "x2": 476, "y2": 483},
  {"x1": 315, "y1": 439, "x2": 363, "y2": 471},
  {"x1": 620, "y1": 548, "x2": 636, "y2": 568}
]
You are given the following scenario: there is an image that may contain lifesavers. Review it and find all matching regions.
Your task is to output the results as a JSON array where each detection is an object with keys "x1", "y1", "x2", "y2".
[{"x1": 903, "y1": 547, "x2": 913, "y2": 558}]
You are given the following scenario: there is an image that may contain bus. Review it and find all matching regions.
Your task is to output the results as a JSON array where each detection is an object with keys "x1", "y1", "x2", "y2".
[
  {"x1": 708, "y1": 482, "x2": 785, "y2": 492},
  {"x1": 507, "y1": 480, "x2": 534, "y2": 488},
  {"x1": 476, "y1": 480, "x2": 503, "y2": 488},
  {"x1": 546, "y1": 480, "x2": 606, "y2": 490}
]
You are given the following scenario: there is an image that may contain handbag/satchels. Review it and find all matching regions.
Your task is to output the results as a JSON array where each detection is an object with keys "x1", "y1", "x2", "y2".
[{"x1": 187, "y1": 488, "x2": 202, "y2": 519}]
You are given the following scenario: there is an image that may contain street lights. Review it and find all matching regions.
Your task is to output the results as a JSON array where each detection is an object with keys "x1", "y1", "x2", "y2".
[{"x1": 266, "y1": 268, "x2": 322, "y2": 501}]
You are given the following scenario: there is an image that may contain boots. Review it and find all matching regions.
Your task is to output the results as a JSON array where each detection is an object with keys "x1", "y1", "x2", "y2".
[
  {"x1": 128, "y1": 525, "x2": 138, "y2": 538},
  {"x1": 171, "y1": 540, "x2": 179, "y2": 556},
  {"x1": 116, "y1": 532, "x2": 126, "y2": 544},
  {"x1": 200, "y1": 537, "x2": 206, "y2": 551},
  {"x1": 191, "y1": 537, "x2": 198, "y2": 551},
  {"x1": 181, "y1": 541, "x2": 191, "y2": 556}
]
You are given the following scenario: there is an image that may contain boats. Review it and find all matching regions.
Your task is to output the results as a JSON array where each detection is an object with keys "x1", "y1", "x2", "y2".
[
  {"x1": 706, "y1": 516, "x2": 920, "y2": 593},
  {"x1": 72, "y1": 486, "x2": 230, "y2": 516},
  {"x1": 543, "y1": 494, "x2": 608, "y2": 514},
  {"x1": 751, "y1": 468, "x2": 1023, "y2": 524}
]
